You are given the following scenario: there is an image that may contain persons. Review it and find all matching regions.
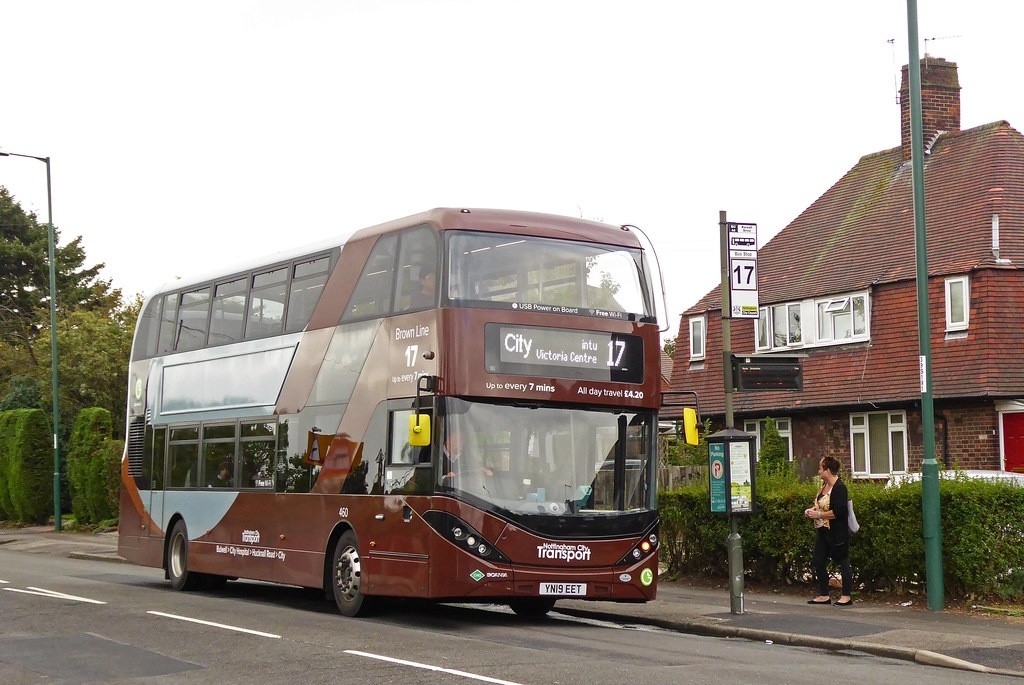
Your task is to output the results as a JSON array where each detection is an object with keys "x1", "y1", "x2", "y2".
[
  {"x1": 805, "y1": 457, "x2": 855, "y2": 606},
  {"x1": 209, "y1": 462, "x2": 235, "y2": 488},
  {"x1": 441, "y1": 429, "x2": 494, "y2": 483}
]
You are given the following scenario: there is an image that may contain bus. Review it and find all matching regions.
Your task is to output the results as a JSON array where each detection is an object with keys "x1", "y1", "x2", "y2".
[{"x1": 116, "y1": 207, "x2": 701, "y2": 616}]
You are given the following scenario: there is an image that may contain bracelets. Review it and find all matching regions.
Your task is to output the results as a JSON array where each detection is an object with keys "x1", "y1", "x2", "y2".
[{"x1": 817, "y1": 511, "x2": 822, "y2": 519}]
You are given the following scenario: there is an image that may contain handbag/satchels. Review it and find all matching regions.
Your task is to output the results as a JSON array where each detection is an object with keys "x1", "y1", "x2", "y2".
[{"x1": 848, "y1": 499, "x2": 860, "y2": 533}]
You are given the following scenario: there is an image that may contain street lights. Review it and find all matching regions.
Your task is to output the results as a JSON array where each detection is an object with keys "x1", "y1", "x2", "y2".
[{"x1": 0, "y1": 150, "x2": 63, "y2": 532}]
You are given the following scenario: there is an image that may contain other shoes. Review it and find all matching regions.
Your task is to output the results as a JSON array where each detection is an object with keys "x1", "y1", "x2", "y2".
[
  {"x1": 833, "y1": 599, "x2": 852, "y2": 606},
  {"x1": 807, "y1": 599, "x2": 831, "y2": 604}
]
